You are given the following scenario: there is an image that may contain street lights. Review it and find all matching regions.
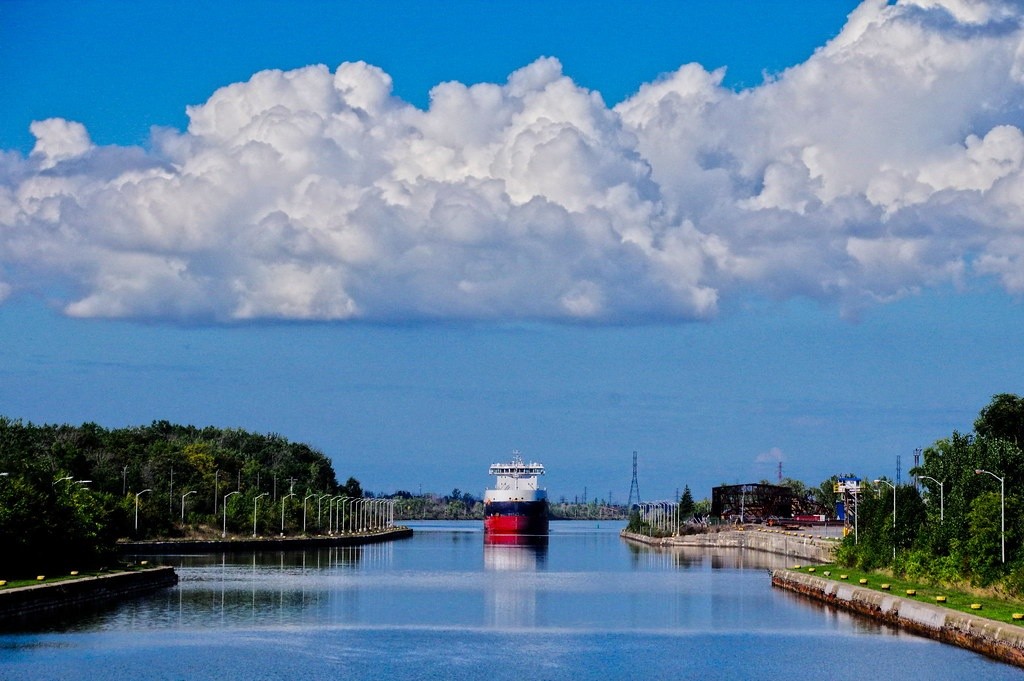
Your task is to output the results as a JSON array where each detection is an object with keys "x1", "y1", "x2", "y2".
[
  {"x1": 975, "y1": 469, "x2": 1005, "y2": 564},
  {"x1": 355, "y1": 498, "x2": 401, "y2": 534},
  {"x1": 304, "y1": 494, "x2": 318, "y2": 532},
  {"x1": 253, "y1": 492, "x2": 269, "y2": 538},
  {"x1": 636, "y1": 499, "x2": 680, "y2": 538},
  {"x1": 918, "y1": 475, "x2": 943, "y2": 527},
  {"x1": 349, "y1": 498, "x2": 360, "y2": 533},
  {"x1": 329, "y1": 495, "x2": 342, "y2": 535},
  {"x1": 222, "y1": 491, "x2": 240, "y2": 538},
  {"x1": 342, "y1": 497, "x2": 354, "y2": 533},
  {"x1": 874, "y1": 479, "x2": 896, "y2": 561},
  {"x1": 335, "y1": 497, "x2": 349, "y2": 533},
  {"x1": 318, "y1": 494, "x2": 332, "y2": 528},
  {"x1": 182, "y1": 491, "x2": 197, "y2": 525},
  {"x1": 135, "y1": 489, "x2": 152, "y2": 530},
  {"x1": 280, "y1": 494, "x2": 296, "y2": 537}
]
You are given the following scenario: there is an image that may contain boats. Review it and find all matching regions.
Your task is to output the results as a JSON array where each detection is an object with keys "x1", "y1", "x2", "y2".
[{"x1": 484, "y1": 448, "x2": 550, "y2": 549}]
